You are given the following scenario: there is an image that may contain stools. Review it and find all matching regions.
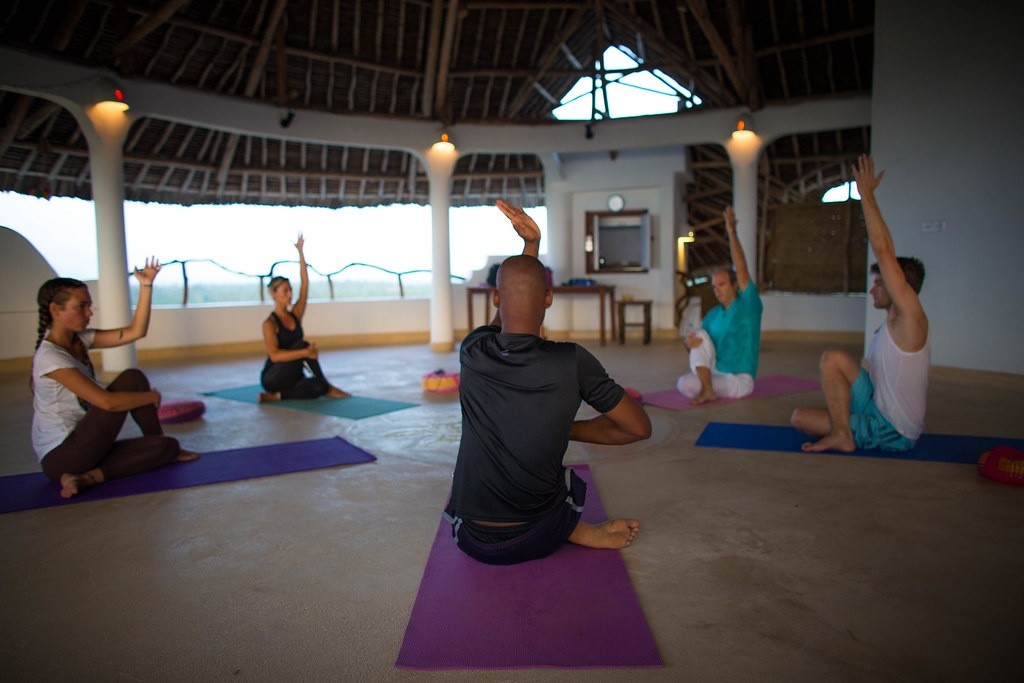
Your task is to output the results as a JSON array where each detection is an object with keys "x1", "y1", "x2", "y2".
[{"x1": 616, "y1": 299, "x2": 653, "y2": 345}]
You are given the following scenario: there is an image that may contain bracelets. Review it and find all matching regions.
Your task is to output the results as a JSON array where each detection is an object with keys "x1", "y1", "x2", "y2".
[{"x1": 140, "y1": 284, "x2": 152, "y2": 288}]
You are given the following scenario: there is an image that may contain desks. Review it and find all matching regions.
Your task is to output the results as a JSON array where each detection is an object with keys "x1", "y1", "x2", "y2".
[{"x1": 466, "y1": 284, "x2": 616, "y2": 346}]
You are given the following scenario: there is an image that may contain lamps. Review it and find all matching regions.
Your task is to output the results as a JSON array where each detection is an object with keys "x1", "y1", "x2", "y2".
[
  {"x1": 431, "y1": 127, "x2": 456, "y2": 151},
  {"x1": 732, "y1": 106, "x2": 756, "y2": 139},
  {"x1": 92, "y1": 74, "x2": 130, "y2": 112}
]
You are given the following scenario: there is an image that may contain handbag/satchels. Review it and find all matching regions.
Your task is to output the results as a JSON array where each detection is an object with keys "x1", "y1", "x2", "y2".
[{"x1": 424, "y1": 369, "x2": 460, "y2": 394}]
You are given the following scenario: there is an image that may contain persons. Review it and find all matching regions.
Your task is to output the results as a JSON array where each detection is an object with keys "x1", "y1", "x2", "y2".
[
  {"x1": 677, "y1": 204, "x2": 765, "y2": 405},
  {"x1": 28, "y1": 256, "x2": 201, "y2": 498},
  {"x1": 441, "y1": 198, "x2": 652, "y2": 566},
  {"x1": 790, "y1": 155, "x2": 931, "y2": 454},
  {"x1": 259, "y1": 232, "x2": 353, "y2": 402}
]
